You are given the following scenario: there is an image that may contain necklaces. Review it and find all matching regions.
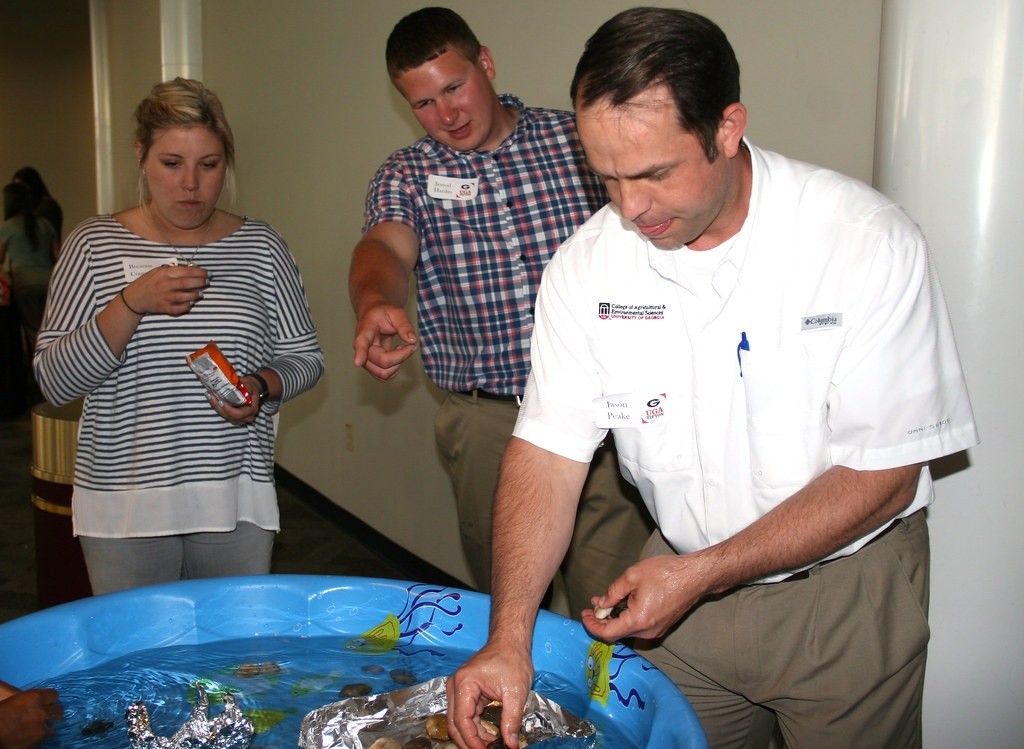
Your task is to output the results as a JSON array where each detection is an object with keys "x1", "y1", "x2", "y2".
[{"x1": 154, "y1": 218, "x2": 209, "y2": 268}]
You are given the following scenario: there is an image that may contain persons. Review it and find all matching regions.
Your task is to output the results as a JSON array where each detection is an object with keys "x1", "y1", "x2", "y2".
[
  {"x1": 443, "y1": 7, "x2": 981, "y2": 748},
  {"x1": 26, "y1": 76, "x2": 322, "y2": 595},
  {"x1": 349, "y1": 5, "x2": 659, "y2": 624},
  {"x1": 1, "y1": 167, "x2": 62, "y2": 353},
  {"x1": 0, "y1": 679, "x2": 64, "y2": 749}
]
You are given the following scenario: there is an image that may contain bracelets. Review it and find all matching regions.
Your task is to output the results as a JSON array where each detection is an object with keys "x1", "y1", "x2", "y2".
[
  {"x1": 244, "y1": 372, "x2": 270, "y2": 401},
  {"x1": 121, "y1": 288, "x2": 146, "y2": 315}
]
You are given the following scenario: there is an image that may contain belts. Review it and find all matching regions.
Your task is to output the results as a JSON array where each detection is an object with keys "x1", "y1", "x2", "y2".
[
  {"x1": 458, "y1": 389, "x2": 523, "y2": 406},
  {"x1": 781, "y1": 517, "x2": 903, "y2": 582}
]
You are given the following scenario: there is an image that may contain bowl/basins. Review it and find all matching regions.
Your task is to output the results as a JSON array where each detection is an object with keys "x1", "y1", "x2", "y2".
[{"x1": 0, "y1": 575, "x2": 706, "y2": 749}]
